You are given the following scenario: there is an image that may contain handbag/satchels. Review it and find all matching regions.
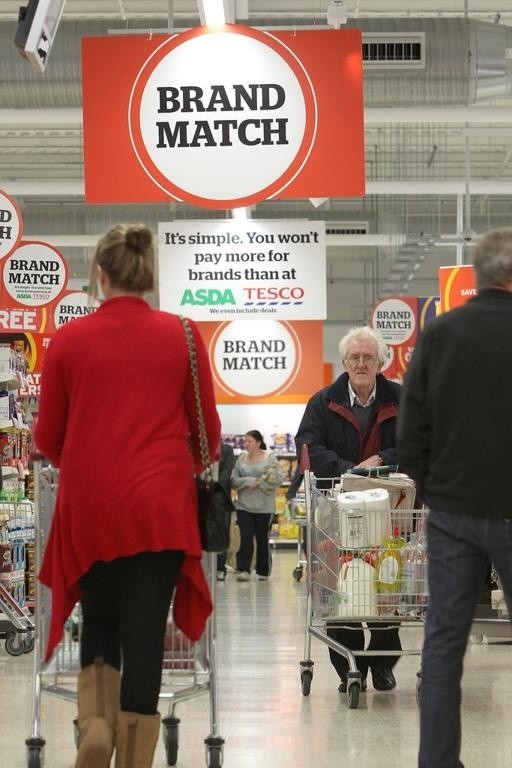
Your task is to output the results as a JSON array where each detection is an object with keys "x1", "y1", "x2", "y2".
[{"x1": 196, "y1": 479, "x2": 235, "y2": 552}]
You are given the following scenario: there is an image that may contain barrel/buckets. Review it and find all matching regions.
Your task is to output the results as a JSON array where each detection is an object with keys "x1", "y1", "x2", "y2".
[
  {"x1": 402, "y1": 533, "x2": 428, "y2": 604},
  {"x1": 337, "y1": 554, "x2": 378, "y2": 618}
]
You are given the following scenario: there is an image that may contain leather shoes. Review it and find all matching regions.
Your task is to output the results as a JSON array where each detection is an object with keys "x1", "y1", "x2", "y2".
[{"x1": 338, "y1": 668, "x2": 396, "y2": 693}]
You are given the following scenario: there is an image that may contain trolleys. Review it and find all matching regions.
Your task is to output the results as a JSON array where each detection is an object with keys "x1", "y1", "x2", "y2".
[
  {"x1": 288, "y1": 491, "x2": 311, "y2": 581},
  {"x1": 299, "y1": 463, "x2": 431, "y2": 709},
  {"x1": 0, "y1": 498, "x2": 37, "y2": 656},
  {"x1": 469, "y1": 614, "x2": 512, "y2": 644},
  {"x1": 17, "y1": 440, "x2": 226, "y2": 768}
]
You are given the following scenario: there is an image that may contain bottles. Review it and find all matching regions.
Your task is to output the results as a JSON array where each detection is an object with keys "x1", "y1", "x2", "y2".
[
  {"x1": 0, "y1": 342, "x2": 34, "y2": 380},
  {"x1": 62, "y1": 619, "x2": 79, "y2": 641},
  {"x1": 377, "y1": 525, "x2": 427, "y2": 618}
]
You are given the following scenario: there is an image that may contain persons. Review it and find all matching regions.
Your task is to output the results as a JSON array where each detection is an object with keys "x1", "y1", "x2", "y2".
[
  {"x1": 30, "y1": 223, "x2": 226, "y2": 768},
  {"x1": 393, "y1": 221, "x2": 510, "y2": 768},
  {"x1": 229, "y1": 429, "x2": 284, "y2": 582},
  {"x1": 294, "y1": 326, "x2": 421, "y2": 694},
  {"x1": 217, "y1": 442, "x2": 234, "y2": 580},
  {"x1": 285, "y1": 462, "x2": 307, "y2": 558}
]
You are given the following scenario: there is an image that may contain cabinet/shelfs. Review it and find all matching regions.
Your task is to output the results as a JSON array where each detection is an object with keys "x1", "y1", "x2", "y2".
[
  {"x1": 1, "y1": 343, "x2": 56, "y2": 633},
  {"x1": 219, "y1": 433, "x2": 308, "y2": 550}
]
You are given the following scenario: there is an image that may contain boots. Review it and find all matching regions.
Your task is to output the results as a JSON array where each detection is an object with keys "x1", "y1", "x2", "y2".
[{"x1": 76, "y1": 656, "x2": 160, "y2": 768}]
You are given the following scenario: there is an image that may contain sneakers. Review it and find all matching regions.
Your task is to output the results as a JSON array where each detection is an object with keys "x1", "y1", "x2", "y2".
[{"x1": 217, "y1": 571, "x2": 268, "y2": 581}]
